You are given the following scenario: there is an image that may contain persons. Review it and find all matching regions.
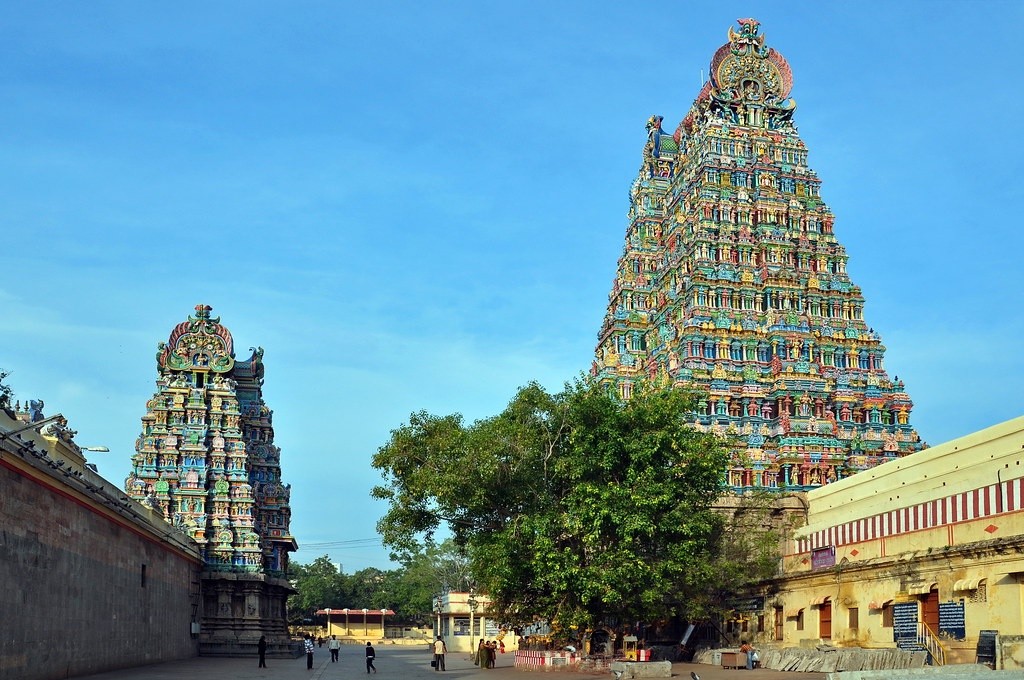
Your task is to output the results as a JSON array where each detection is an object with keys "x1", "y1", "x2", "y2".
[
  {"x1": 318, "y1": 636, "x2": 323, "y2": 647},
  {"x1": 328, "y1": 634, "x2": 340, "y2": 663},
  {"x1": 740, "y1": 641, "x2": 757, "y2": 670},
  {"x1": 433, "y1": 636, "x2": 448, "y2": 672},
  {"x1": 366, "y1": 641, "x2": 378, "y2": 674},
  {"x1": 258, "y1": 635, "x2": 268, "y2": 668},
  {"x1": 304, "y1": 634, "x2": 315, "y2": 670},
  {"x1": 478, "y1": 639, "x2": 498, "y2": 670}
]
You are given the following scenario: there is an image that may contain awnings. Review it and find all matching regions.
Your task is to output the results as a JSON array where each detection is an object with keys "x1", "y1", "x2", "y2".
[
  {"x1": 953, "y1": 577, "x2": 988, "y2": 592},
  {"x1": 998, "y1": 568, "x2": 1024, "y2": 576},
  {"x1": 809, "y1": 595, "x2": 831, "y2": 606},
  {"x1": 867, "y1": 599, "x2": 893, "y2": 610},
  {"x1": 909, "y1": 581, "x2": 938, "y2": 595},
  {"x1": 786, "y1": 608, "x2": 805, "y2": 617}
]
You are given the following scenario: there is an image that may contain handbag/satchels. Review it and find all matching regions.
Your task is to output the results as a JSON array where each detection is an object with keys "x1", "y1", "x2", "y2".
[{"x1": 431, "y1": 661, "x2": 435, "y2": 667}]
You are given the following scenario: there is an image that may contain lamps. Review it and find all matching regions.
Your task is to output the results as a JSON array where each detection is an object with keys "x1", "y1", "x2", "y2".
[{"x1": 82, "y1": 446, "x2": 109, "y2": 452}]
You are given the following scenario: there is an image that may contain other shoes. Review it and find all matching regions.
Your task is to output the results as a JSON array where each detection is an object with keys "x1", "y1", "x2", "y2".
[
  {"x1": 483, "y1": 668, "x2": 487, "y2": 669},
  {"x1": 373, "y1": 668, "x2": 377, "y2": 674}
]
